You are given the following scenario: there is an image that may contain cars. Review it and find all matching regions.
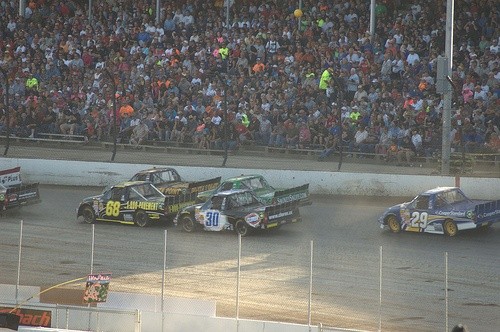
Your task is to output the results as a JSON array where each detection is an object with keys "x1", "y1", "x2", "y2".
[
  {"x1": 378, "y1": 186, "x2": 500, "y2": 237},
  {"x1": 1, "y1": 180, "x2": 43, "y2": 209},
  {"x1": 197, "y1": 174, "x2": 310, "y2": 216},
  {"x1": 172, "y1": 189, "x2": 302, "y2": 236},
  {"x1": 76, "y1": 180, "x2": 198, "y2": 227},
  {"x1": 128, "y1": 166, "x2": 221, "y2": 209}
]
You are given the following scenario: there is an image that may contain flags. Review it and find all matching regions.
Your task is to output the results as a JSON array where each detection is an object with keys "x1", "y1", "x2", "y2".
[{"x1": 82, "y1": 273, "x2": 112, "y2": 303}]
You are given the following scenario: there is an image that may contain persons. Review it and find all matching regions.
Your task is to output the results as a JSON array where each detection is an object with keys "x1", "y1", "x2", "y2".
[{"x1": 0, "y1": 0, "x2": 499, "y2": 164}]
